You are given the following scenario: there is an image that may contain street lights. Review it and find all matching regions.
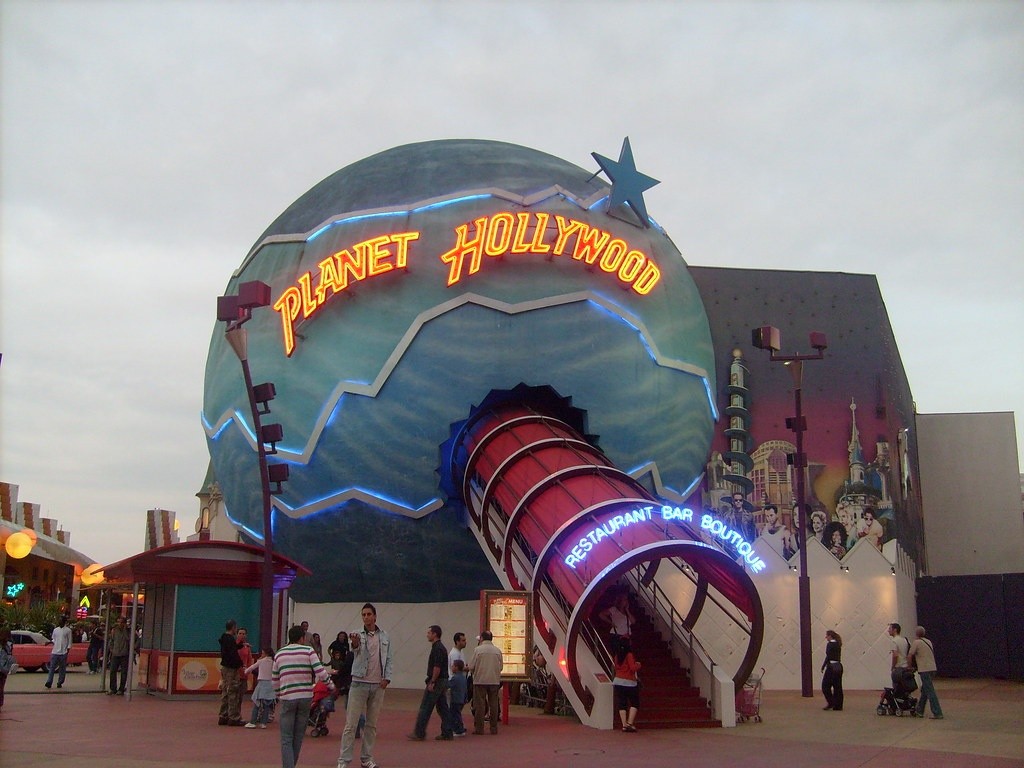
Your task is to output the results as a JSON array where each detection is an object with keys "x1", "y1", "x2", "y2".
[
  {"x1": 752, "y1": 325, "x2": 829, "y2": 698},
  {"x1": 217, "y1": 280, "x2": 288, "y2": 693}
]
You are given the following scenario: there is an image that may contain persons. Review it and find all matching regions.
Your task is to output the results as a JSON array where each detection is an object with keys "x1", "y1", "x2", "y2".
[
  {"x1": 724, "y1": 493, "x2": 756, "y2": 545},
  {"x1": 907, "y1": 626, "x2": 945, "y2": 719},
  {"x1": 0, "y1": 626, "x2": 13, "y2": 708},
  {"x1": 612, "y1": 637, "x2": 642, "y2": 732},
  {"x1": 218, "y1": 619, "x2": 247, "y2": 726},
  {"x1": 788, "y1": 502, "x2": 883, "y2": 561},
  {"x1": 301, "y1": 621, "x2": 357, "y2": 710},
  {"x1": 406, "y1": 625, "x2": 454, "y2": 740},
  {"x1": 599, "y1": 595, "x2": 635, "y2": 656},
  {"x1": 45, "y1": 616, "x2": 72, "y2": 688},
  {"x1": 446, "y1": 631, "x2": 504, "y2": 737},
  {"x1": 70, "y1": 615, "x2": 142, "y2": 696},
  {"x1": 759, "y1": 504, "x2": 790, "y2": 563},
  {"x1": 821, "y1": 630, "x2": 844, "y2": 711},
  {"x1": 244, "y1": 647, "x2": 276, "y2": 728},
  {"x1": 271, "y1": 626, "x2": 337, "y2": 768},
  {"x1": 888, "y1": 623, "x2": 917, "y2": 716},
  {"x1": 235, "y1": 628, "x2": 253, "y2": 720},
  {"x1": 338, "y1": 603, "x2": 393, "y2": 768}
]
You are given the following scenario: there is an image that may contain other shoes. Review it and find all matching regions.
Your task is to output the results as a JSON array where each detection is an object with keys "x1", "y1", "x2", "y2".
[
  {"x1": 406, "y1": 735, "x2": 425, "y2": 741},
  {"x1": 116, "y1": 691, "x2": 124, "y2": 695},
  {"x1": 218, "y1": 717, "x2": 230, "y2": 725},
  {"x1": 245, "y1": 722, "x2": 256, "y2": 729},
  {"x1": 453, "y1": 732, "x2": 465, "y2": 736},
  {"x1": 338, "y1": 760, "x2": 347, "y2": 768},
  {"x1": 361, "y1": 761, "x2": 379, "y2": 768},
  {"x1": 228, "y1": 720, "x2": 247, "y2": 726},
  {"x1": 622, "y1": 723, "x2": 637, "y2": 732},
  {"x1": 105, "y1": 690, "x2": 114, "y2": 695},
  {"x1": 436, "y1": 735, "x2": 453, "y2": 740},
  {"x1": 258, "y1": 723, "x2": 266, "y2": 728}
]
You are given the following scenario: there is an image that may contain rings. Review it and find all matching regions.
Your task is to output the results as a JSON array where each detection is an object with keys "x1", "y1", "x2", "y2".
[{"x1": 359, "y1": 636, "x2": 360, "y2": 639}]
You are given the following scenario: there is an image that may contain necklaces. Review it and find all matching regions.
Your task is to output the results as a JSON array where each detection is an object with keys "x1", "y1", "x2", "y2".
[{"x1": 367, "y1": 630, "x2": 377, "y2": 637}]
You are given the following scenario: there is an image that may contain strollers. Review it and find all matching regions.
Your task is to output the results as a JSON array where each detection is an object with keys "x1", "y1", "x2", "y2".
[
  {"x1": 877, "y1": 664, "x2": 918, "y2": 717},
  {"x1": 308, "y1": 680, "x2": 338, "y2": 737}
]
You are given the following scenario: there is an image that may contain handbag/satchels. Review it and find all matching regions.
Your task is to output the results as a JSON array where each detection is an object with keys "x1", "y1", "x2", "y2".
[{"x1": 907, "y1": 646, "x2": 917, "y2": 672}]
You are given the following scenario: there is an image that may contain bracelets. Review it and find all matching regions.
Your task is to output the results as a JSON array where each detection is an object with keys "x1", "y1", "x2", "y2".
[
  {"x1": 386, "y1": 680, "x2": 390, "y2": 684},
  {"x1": 429, "y1": 680, "x2": 435, "y2": 684},
  {"x1": 333, "y1": 670, "x2": 336, "y2": 675}
]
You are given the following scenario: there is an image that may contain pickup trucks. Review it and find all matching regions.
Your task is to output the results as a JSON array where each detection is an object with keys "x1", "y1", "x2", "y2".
[{"x1": 11, "y1": 630, "x2": 101, "y2": 672}]
